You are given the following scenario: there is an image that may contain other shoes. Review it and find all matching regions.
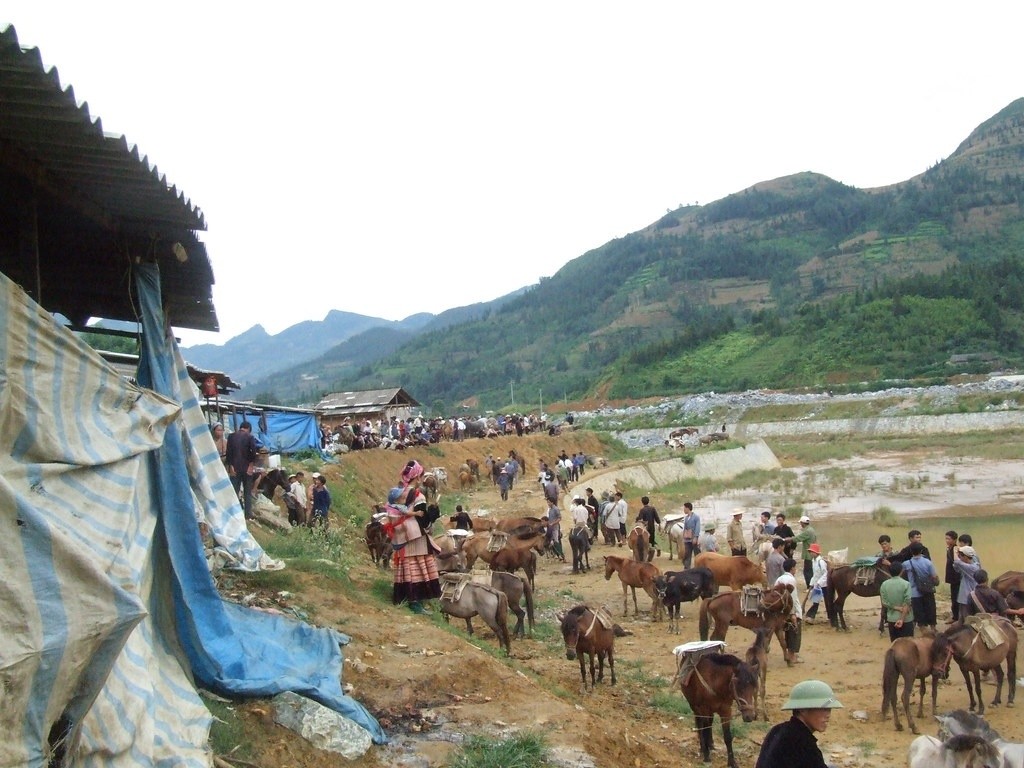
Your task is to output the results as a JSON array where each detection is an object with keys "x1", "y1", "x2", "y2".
[
  {"x1": 787, "y1": 655, "x2": 804, "y2": 663},
  {"x1": 410, "y1": 601, "x2": 432, "y2": 616},
  {"x1": 804, "y1": 618, "x2": 815, "y2": 625},
  {"x1": 944, "y1": 619, "x2": 955, "y2": 624},
  {"x1": 604, "y1": 540, "x2": 627, "y2": 548},
  {"x1": 657, "y1": 548, "x2": 661, "y2": 556}
]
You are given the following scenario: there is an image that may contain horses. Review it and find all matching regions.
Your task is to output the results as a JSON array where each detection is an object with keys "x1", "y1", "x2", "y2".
[
  {"x1": 423, "y1": 465, "x2": 448, "y2": 499},
  {"x1": 367, "y1": 513, "x2": 1024, "y2": 768},
  {"x1": 507, "y1": 450, "x2": 525, "y2": 474},
  {"x1": 664, "y1": 427, "x2": 729, "y2": 450},
  {"x1": 458, "y1": 459, "x2": 480, "y2": 492},
  {"x1": 584, "y1": 455, "x2": 607, "y2": 469}
]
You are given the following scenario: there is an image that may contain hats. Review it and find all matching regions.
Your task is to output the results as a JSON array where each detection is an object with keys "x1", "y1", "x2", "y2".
[
  {"x1": 500, "y1": 470, "x2": 508, "y2": 473},
  {"x1": 312, "y1": 472, "x2": 321, "y2": 478},
  {"x1": 806, "y1": 544, "x2": 821, "y2": 554},
  {"x1": 601, "y1": 491, "x2": 610, "y2": 499},
  {"x1": 730, "y1": 508, "x2": 745, "y2": 515},
  {"x1": 389, "y1": 486, "x2": 406, "y2": 503},
  {"x1": 418, "y1": 415, "x2": 423, "y2": 418},
  {"x1": 958, "y1": 546, "x2": 975, "y2": 558},
  {"x1": 289, "y1": 474, "x2": 297, "y2": 478},
  {"x1": 798, "y1": 515, "x2": 810, "y2": 523}
]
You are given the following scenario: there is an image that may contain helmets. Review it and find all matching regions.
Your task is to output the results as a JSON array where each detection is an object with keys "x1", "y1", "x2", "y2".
[
  {"x1": 781, "y1": 680, "x2": 844, "y2": 709},
  {"x1": 704, "y1": 522, "x2": 716, "y2": 530}
]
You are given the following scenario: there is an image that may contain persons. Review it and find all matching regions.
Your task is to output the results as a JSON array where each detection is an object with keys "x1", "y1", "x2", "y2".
[
  {"x1": 728, "y1": 509, "x2": 827, "y2": 662},
  {"x1": 705, "y1": 524, "x2": 720, "y2": 551},
  {"x1": 320, "y1": 409, "x2": 546, "y2": 450},
  {"x1": 450, "y1": 505, "x2": 473, "y2": 530},
  {"x1": 635, "y1": 497, "x2": 661, "y2": 557},
  {"x1": 878, "y1": 529, "x2": 1024, "y2": 681},
  {"x1": 388, "y1": 461, "x2": 441, "y2": 615},
  {"x1": 570, "y1": 487, "x2": 626, "y2": 547},
  {"x1": 538, "y1": 450, "x2": 585, "y2": 503},
  {"x1": 485, "y1": 454, "x2": 518, "y2": 501},
  {"x1": 683, "y1": 503, "x2": 700, "y2": 569},
  {"x1": 251, "y1": 472, "x2": 261, "y2": 500},
  {"x1": 212, "y1": 422, "x2": 227, "y2": 460},
  {"x1": 541, "y1": 497, "x2": 563, "y2": 561},
  {"x1": 755, "y1": 680, "x2": 843, "y2": 768},
  {"x1": 307, "y1": 473, "x2": 331, "y2": 530},
  {"x1": 566, "y1": 413, "x2": 573, "y2": 424},
  {"x1": 226, "y1": 422, "x2": 256, "y2": 520},
  {"x1": 549, "y1": 425, "x2": 561, "y2": 435},
  {"x1": 722, "y1": 423, "x2": 726, "y2": 432},
  {"x1": 284, "y1": 472, "x2": 307, "y2": 525}
]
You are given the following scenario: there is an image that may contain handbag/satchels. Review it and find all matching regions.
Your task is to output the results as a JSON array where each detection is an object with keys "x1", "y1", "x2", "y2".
[
  {"x1": 683, "y1": 529, "x2": 694, "y2": 539},
  {"x1": 912, "y1": 573, "x2": 937, "y2": 594}
]
